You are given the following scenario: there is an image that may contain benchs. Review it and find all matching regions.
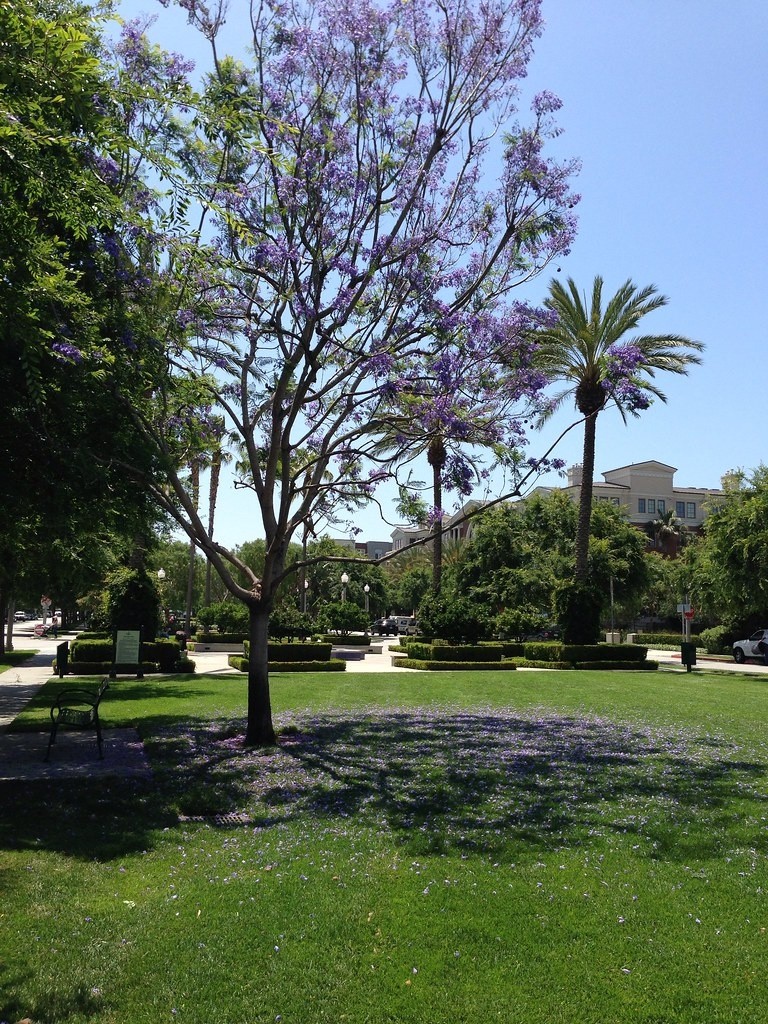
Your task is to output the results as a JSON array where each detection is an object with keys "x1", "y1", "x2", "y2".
[{"x1": 42, "y1": 676, "x2": 110, "y2": 764}]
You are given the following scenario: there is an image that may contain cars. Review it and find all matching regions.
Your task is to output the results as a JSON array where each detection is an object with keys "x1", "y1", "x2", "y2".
[
  {"x1": 55, "y1": 611, "x2": 61, "y2": 616},
  {"x1": 369, "y1": 619, "x2": 398, "y2": 636},
  {"x1": 15, "y1": 612, "x2": 25, "y2": 622},
  {"x1": 405, "y1": 621, "x2": 423, "y2": 636},
  {"x1": 732, "y1": 629, "x2": 768, "y2": 664}
]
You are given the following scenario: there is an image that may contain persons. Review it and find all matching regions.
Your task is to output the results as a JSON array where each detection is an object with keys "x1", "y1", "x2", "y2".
[
  {"x1": 51, "y1": 618, "x2": 57, "y2": 638},
  {"x1": 761, "y1": 625, "x2": 768, "y2": 666},
  {"x1": 160, "y1": 627, "x2": 169, "y2": 638},
  {"x1": 175, "y1": 628, "x2": 186, "y2": 651}
]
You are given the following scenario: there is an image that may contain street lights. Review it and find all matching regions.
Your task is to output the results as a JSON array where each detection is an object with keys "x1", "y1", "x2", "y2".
[
  {"x1": 341, "y1": 572, "x2": 348, "y2": 602},
  {"x1": 304, "y1": 580, "x2": 308, "y2": 612},
  {"x1": 364, "y1": 584, "x2": 369, "y2": 612}
]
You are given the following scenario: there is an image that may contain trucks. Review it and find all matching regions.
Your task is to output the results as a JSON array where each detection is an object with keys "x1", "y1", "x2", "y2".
[{"x1": 388, "y1": 616, "x2": 413, "y2": 631}]
